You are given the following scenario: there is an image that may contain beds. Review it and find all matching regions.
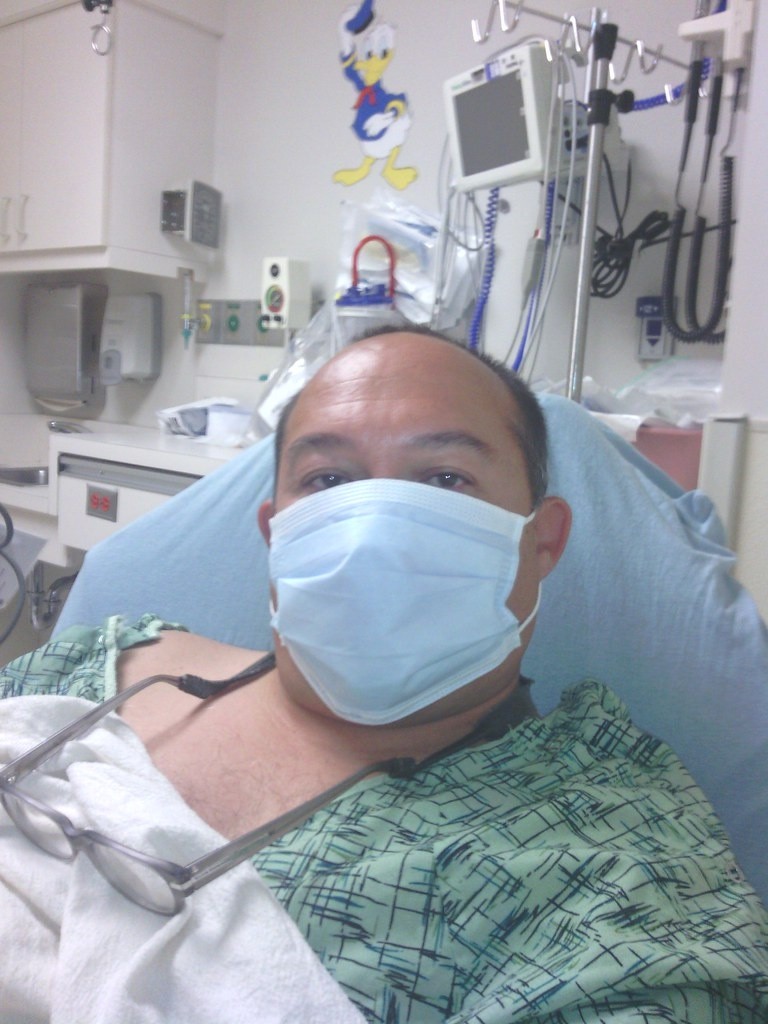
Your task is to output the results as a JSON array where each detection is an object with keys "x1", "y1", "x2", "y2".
[{"x1": 51, "y1": 389, "x2": 768, "y2": 913}]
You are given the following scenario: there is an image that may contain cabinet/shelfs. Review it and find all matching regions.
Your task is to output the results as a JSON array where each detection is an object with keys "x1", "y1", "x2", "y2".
[{"x1": 0, "y1": 0, "x2": 223, "y2": 283}]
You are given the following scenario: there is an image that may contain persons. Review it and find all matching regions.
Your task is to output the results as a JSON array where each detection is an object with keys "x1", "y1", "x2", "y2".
[{"x1": 0, "y1": 322, "x2": 768, "y2": 1024}]
